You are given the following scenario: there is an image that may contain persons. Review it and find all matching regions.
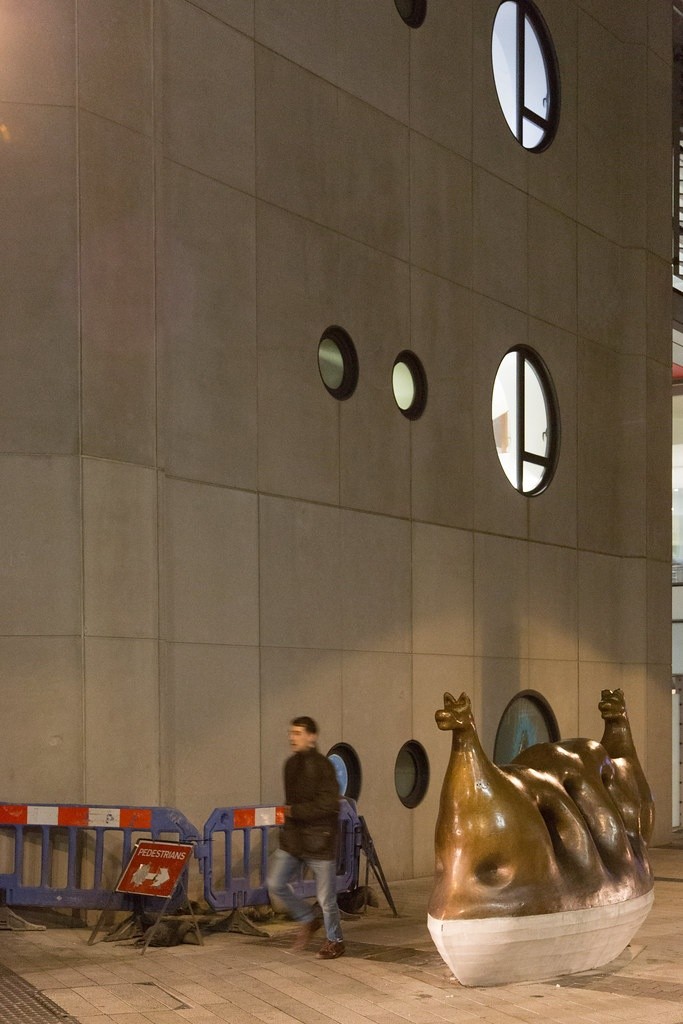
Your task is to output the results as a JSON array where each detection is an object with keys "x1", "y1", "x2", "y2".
[{"x1": 264, "y1": 716, "x2": 346, "y2": 959}]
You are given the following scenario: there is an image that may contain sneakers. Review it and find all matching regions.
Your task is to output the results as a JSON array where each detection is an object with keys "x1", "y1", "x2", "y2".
[
  {"x1": 294, "y1": 918, "x2": 323, "y2": 950},
  {"x1": 315, "y1": 940, "x2": 346, "y2": 959}
]
